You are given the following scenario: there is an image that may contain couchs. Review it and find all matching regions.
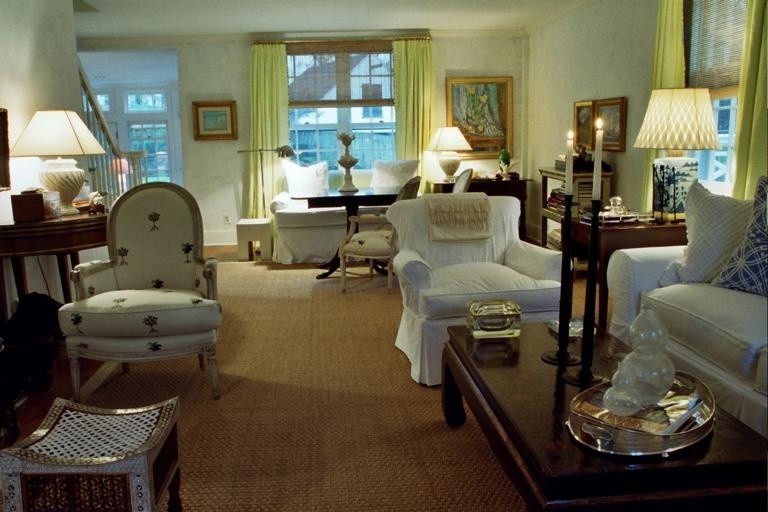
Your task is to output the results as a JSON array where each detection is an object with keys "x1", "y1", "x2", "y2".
[
  {"x1": 387, "y1": 191, "x2": 573, "y2": 386},
  {"x1": 271, "y1": 169, "x2": 422, "y2": 265},
  {"x1": 605, "y1": 245, "x2": 768, "y2": 438}
]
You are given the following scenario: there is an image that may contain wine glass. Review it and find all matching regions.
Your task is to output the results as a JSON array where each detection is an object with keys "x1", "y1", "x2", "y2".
[{"x1": 610, "y1": 196, "x2": 622, "y2": 216}]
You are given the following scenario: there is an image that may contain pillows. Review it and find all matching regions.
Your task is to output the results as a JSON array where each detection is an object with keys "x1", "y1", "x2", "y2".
[
  {"x1": 711, "y1": 176, "x2": 768, "y2": 297},
  {"x1": 286, "y1": 161, "x2": 330, "y2": 191},
  {"x1": 371, "y1": 159, "x2": 420, "y2": 186},
  {"x1": 659, "y1": 181, "x2": 754, "y2": 287}
]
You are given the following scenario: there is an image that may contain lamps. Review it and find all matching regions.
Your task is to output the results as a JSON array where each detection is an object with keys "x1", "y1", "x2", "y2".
[
  {"x1": 237, "y1": 146, "x2": 297, "y2": 254},
  {"x1": 9, "y1": 110, "x2": 106, "y2": 216},
  {"x1": 106, "y1": 158, "x2": 133, "y2": 195},
  {"x1": 426, "y1": 127, "x2": 472, "y2": 181},
  {"x1": 634, "y1": 87, "x2": 722, "y2": 222}
]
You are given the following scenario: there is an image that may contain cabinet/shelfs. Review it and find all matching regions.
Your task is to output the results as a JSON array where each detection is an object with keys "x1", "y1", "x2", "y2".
[{"x1": 537, "y1": 166, "x2": 615, "y2": 285}]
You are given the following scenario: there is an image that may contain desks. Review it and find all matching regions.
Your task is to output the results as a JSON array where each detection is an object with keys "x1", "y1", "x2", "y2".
[
  {"x1": 1, "y1": 209, "x2": 108, "y2": 327},
  {"x1": 433, "y1": 178, "x2": 535, "y2": 240},
  {"x1": 570, "y1": 218, "x2": 687, "y2": 329},
  {"x1": 291, "y1": 186, "x2": 401, "y2": 279}
]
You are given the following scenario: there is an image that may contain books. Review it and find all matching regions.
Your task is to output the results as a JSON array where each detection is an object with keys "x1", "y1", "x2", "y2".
[
  {"x1": 548, "y1": 182, "x2": 593, "y2": 213},
  {"x1": 547, "y1": 229, "x2": 562, "y2": 250}
]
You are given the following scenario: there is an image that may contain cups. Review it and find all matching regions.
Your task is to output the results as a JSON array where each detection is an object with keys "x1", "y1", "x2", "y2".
[{"x1": 43, "y1": 192, "x2": 61, "y2": 218}]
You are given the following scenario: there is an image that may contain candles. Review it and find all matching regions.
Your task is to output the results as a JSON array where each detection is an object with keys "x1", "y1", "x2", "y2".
[
  {"x1": 565, "y1": 130, "x2": 574, "y2": 195},
  {"x1": 591, "y1": 118, "x2": 603, "y2": 201}
]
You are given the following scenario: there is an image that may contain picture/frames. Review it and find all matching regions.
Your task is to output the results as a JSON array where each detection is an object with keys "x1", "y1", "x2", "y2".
[
  {"x1": 192, "y1": 100, "x2": 238, "y2": 141},
  {"x1": 593, "y1": 97, "x2": 628, "y2": 152},
  {"x1": 445, "y1": 76, "x2": 514, "y2": 160},
  {"x1": 574, "y1": 101, "x2": 592, "y2": 151}
]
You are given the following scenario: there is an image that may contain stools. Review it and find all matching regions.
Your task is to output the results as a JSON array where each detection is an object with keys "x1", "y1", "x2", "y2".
[
  {"x1": 235, "y1": 217, "x2": 274, "y2": 262},
  {"x1": 1, "y1": 397, "x2": 180, "y2": 511}
]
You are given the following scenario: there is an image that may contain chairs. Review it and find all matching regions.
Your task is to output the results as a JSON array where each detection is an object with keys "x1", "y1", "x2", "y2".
[
  {"x1": 56, "y1": 181, "x2": 223, "y2": 400},
  {"x1": 451, "y1": 169, "x2": 473, "y2": 193},
  {"x1": 339, "y1": 177, "x2": 421, "y2": 294}
]
